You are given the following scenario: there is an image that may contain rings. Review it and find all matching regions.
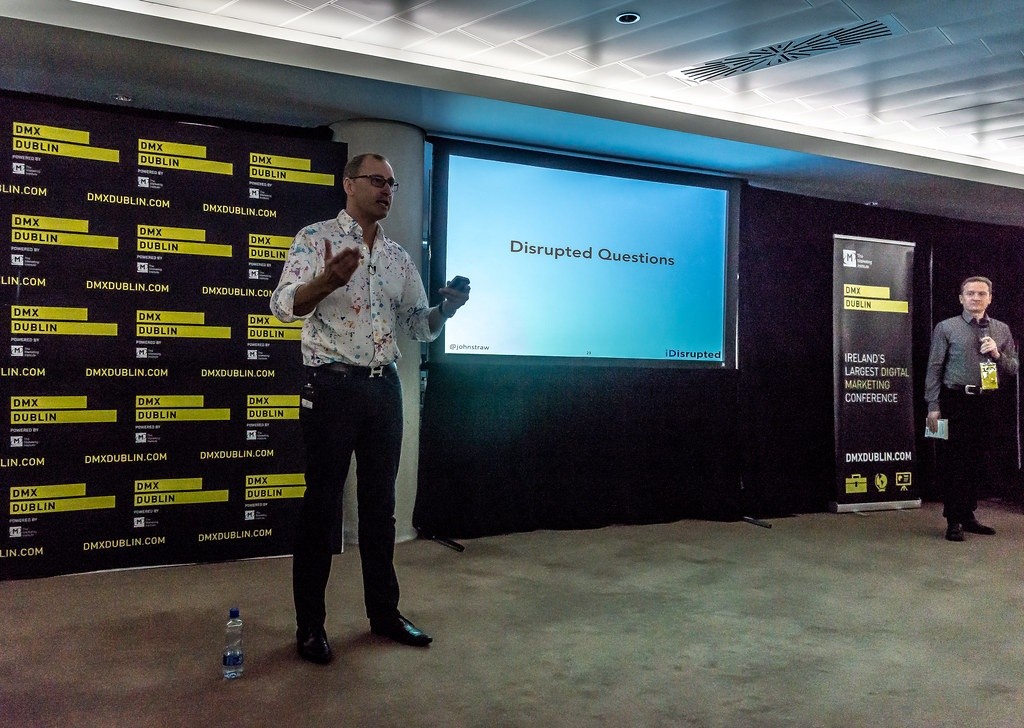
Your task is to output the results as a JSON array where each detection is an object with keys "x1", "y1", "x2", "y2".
[{"x1": 987, "y1": 342, "x2": 990, "y2": 345}]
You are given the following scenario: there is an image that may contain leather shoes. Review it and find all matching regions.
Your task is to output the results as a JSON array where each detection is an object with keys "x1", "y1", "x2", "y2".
[
  {"x1": 296, "y1": 626, "x2": 332, "y2": 664},
  {"x1": 372, "y1": 609, "x2": 433, "y2": 645}
]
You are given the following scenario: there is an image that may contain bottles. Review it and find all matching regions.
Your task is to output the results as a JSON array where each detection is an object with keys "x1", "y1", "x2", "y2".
[{"x1": 221, "y1": 607, "x2": 245, "y2": 679}]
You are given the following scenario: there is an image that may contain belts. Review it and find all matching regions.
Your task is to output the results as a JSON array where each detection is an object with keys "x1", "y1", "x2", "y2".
[
  {"x1": 313, "y1": 361, "x2": 398, "y2": 378},
  {"x1": 947, "y1": 384, "x2": 992, "y2": 396}
]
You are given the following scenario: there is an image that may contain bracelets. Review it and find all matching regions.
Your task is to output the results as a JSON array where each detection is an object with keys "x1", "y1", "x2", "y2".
[{"x1": 439, "y1": 302, "x2": 456, "y2": 318}]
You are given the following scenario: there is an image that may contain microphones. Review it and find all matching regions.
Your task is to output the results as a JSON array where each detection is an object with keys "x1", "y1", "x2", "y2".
[
  {"x1": 374, "y1": 266, "x2": 377, "y2": 275},
  {"x1": 978, "y1": 318, "x2": 990, "y2": 358}
]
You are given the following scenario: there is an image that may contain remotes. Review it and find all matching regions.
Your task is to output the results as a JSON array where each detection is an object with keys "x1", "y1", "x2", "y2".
[{"x1": 446, "y1": 276, "x2": 469, "y2": 292}]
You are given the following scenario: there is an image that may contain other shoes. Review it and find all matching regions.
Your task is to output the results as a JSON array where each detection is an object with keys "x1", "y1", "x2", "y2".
[
  {"x1": 945, "y1": 520, "x2": 964, "y2": 542},
  {"x1": 961, "y1": 518, "x2": 996, "y2": 534}
]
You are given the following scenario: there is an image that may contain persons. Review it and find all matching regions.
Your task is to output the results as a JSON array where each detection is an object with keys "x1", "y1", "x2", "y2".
[
  {"x1": 270, "y1": 152, "x2": 470, "y2": 666},
  {"x1": 924, "y1": 275, "x2": 1019, "y2": 541}
]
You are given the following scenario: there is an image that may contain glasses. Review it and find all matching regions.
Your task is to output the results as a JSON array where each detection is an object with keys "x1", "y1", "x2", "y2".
[{"x1": 350, "y1": 173, "x2": 400, "y2": 191}]
